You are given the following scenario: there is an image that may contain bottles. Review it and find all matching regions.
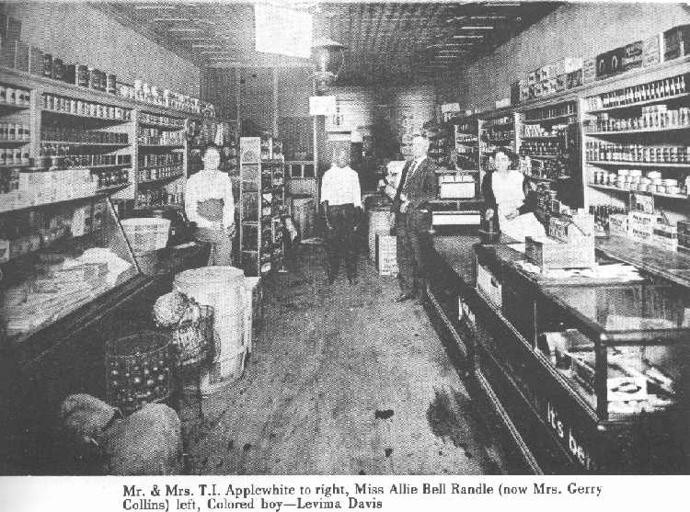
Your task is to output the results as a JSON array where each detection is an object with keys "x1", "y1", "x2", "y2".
[
  {"x1": 598, "y1": 76, "x2": 690, "y2": 131},
  {"x1": 586, "y1": 141, "x2": 643, "y2": 161},
  {"x1": 597, "y1": 205, "x2": 628, "y2": 232}
]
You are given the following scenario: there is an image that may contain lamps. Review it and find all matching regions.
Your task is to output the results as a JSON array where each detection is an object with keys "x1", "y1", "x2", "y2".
[{"x1": 303, "y1": 35, "x2": 346, "y2": 92}]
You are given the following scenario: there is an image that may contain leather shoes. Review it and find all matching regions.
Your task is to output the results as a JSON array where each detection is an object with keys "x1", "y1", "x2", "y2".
[{"x1": 394, "y1": 292, "x2": 430, "y2": 305}]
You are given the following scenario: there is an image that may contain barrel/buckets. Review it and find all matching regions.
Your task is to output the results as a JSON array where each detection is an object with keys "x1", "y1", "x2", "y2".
[
  {"x1": 173, "y1": 265, "x2": 244, "y2": 396},
  {"x1": 368, "y1": 208, "x2": 390, "y2": 263},
  {"x1": 105, "y1": 331, "x2": 171, "y2": 411}
]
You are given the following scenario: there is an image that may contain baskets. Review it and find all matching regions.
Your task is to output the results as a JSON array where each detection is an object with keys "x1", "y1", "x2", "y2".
[{"x1": 152, "y1": 306, "x2": 217, "y2": 370}]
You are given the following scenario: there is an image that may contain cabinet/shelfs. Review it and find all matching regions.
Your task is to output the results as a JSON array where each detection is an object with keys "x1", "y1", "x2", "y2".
[
  {"x1": 0, "y1": 67, "x2": 288, "y2": 281},
  {"x1": 424, "y1": 57, "x2": 689, "y2": 475}
]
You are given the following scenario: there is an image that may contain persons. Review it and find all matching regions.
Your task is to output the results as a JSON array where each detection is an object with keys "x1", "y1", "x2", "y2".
[
  {"x1": 182, "y1": 138, "x2": 237, "y2": 268},
  {"x1": 480, "y1": 146, "x2": 547, "y2": 245},
  {"x1": 387, "y1": 135, "x2": 442, "y2": 302},
  {"x1": 321, "y1": 147, "x2": 365, "y2": 284}
]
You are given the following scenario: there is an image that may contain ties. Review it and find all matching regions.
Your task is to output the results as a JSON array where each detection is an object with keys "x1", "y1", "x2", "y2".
[{"x1": 402, "y1": 161, "x2": 418, "y2": 196}]
